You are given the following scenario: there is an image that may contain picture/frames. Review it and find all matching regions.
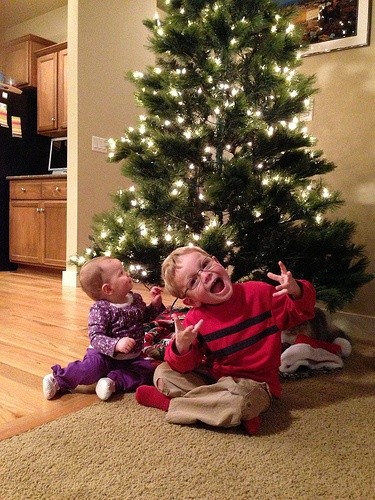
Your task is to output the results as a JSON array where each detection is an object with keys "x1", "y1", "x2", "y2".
[{"x1": 284, "y1": 0, "x2": 372, "y2": 56}]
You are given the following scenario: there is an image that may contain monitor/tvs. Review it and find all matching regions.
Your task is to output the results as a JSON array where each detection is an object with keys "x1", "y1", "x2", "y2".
[{"x1": 48, "y1": 137, "x2": 67, "y2": 174}]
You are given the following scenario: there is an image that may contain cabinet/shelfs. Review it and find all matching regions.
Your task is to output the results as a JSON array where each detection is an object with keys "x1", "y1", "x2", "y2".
[{"x1": 2, "y1": 34, "x2": 68, "y2": 275}]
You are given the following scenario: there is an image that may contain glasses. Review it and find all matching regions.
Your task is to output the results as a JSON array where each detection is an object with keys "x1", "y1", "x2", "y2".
[{"x1": 184, "y1": 255, "x2": 213, "y2": 298}]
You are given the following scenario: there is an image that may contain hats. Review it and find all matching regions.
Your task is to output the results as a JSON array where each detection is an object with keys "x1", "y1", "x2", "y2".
[{"x1": 278, "y1": 334, "x2": 352, "y2": 373}]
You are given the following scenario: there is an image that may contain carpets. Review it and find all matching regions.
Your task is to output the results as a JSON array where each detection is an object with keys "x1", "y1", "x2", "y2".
[{"x1": 1, "y1": 336, "x2": 375, "y2": 499}]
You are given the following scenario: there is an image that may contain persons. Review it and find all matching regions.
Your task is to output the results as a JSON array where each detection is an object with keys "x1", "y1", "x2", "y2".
[
  {"x1": 136, "y1": 247, "x2": 316, "y2": 437},
  {"x1": 43, "y1": 257, "x2": 167, "y2": 401}
]
